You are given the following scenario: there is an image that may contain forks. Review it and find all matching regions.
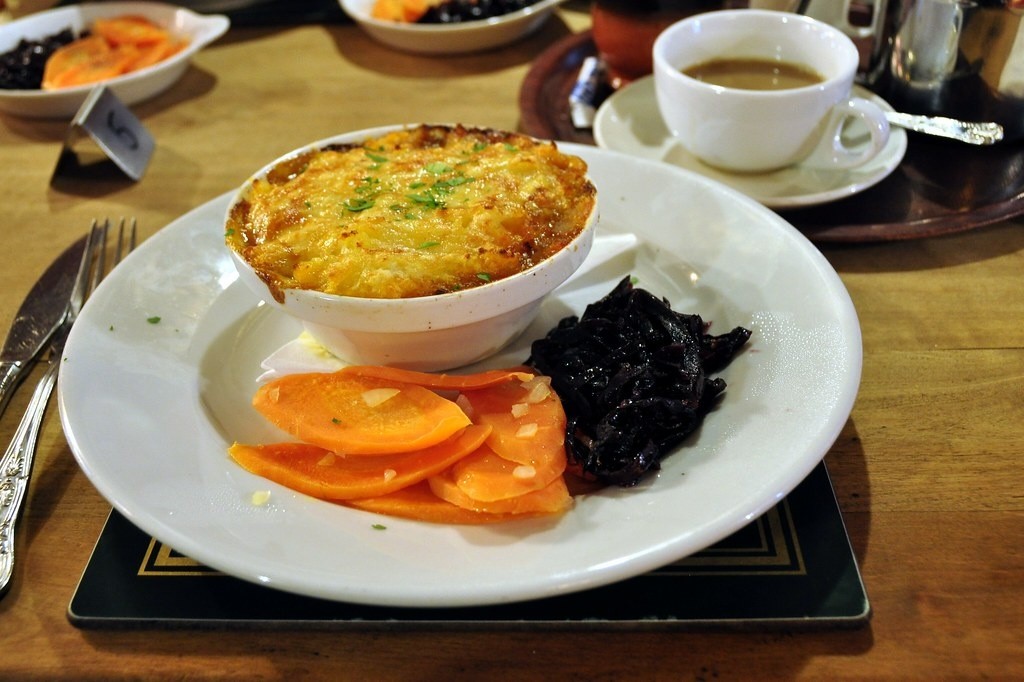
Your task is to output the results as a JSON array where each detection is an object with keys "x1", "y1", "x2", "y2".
[{"x1": 0, "y1": 218, "x2": 142, "y2": 588}]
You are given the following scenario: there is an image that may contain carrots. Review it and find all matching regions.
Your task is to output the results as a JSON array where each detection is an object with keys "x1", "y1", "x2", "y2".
[
  {"x1": 43, "y1": 15, "x2": 186, "y2": 92},
  {"x1": 227, "y1": 363, "x2": 573, "y2": 524},
  {"x1": 372, "y1": 0, "x2": 438, "y2": 26}
]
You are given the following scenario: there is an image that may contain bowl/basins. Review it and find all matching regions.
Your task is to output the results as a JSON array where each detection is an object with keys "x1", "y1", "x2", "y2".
[
  {"x1": 651, "y1": 9, "x2": 860, "y2": 174},
  {"x1": 0, "y1": 3, "x2": 230, "y2": 118},
  {"x1": 336, "y1": 0, "x2": 564, "y2": 55},
  {"x1": 222, "y1": 123, "x2": 600, "y2": 373},
  {"x1": 584, "y1": 0, "x2": 749, "y2": 91}
]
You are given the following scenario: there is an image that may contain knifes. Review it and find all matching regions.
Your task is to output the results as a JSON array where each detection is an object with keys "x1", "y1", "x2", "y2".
[{"x1": 0, "y1": 221, "x2": 112, "y2": 421}]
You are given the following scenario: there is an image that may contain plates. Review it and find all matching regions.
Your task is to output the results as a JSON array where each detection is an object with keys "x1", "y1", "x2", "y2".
[
  {"x1": 592, "y1": 74, "x2": 908, "y2": 206},
  {"x1": 58, "y1": 123, "x2": 866, "y2": 607}
]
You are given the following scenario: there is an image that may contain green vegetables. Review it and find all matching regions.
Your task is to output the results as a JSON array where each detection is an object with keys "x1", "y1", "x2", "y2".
[{"x1": 225, "y1": 137, "x2": 519, "y2": 295}]
